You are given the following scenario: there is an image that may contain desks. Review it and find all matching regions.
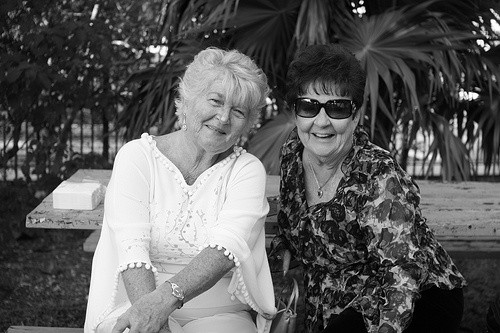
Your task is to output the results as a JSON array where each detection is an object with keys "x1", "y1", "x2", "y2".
[{"x1": 25, "y1": 167, "x2": 500, "y2": 256}]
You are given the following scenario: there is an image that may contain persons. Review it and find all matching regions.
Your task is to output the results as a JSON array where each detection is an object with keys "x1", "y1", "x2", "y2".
[
  {"x1": 270, "y1": 45, "x2": 468, "y2": 333},
  {"x1": 81, "y1": 45, "x2": 278, "y2": 333}
]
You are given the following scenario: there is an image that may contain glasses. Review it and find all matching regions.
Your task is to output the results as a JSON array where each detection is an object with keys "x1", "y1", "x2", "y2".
[{"x1": 292, "y1": 96, "x2": 358, "y2": 119}]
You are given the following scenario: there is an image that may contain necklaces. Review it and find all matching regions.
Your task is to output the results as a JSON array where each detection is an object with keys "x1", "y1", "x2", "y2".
[{"x1": 306, "y1": 150, "x2": 340, "y2": 199}]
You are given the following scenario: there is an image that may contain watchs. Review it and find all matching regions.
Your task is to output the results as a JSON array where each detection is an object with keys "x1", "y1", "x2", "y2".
[{"x1": 164, "y1": 279, "x2": 185, "y2": 309}]
